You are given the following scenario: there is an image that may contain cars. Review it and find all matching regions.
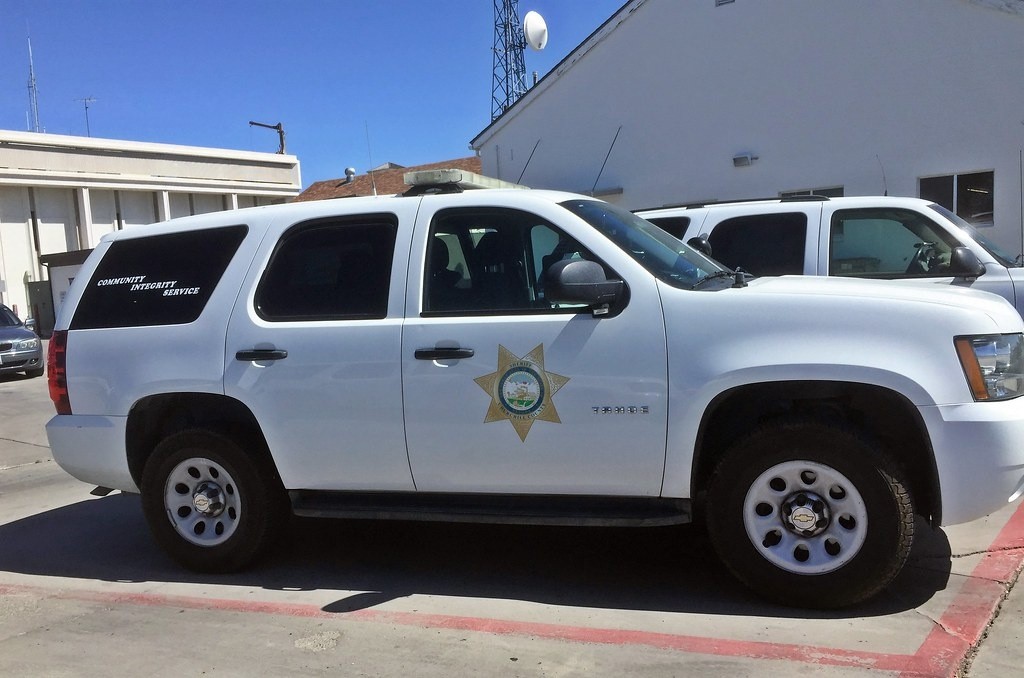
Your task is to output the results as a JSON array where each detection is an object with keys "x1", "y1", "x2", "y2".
[{"x1": 0, "y1": 300, "x2": 43, "y2": 381}]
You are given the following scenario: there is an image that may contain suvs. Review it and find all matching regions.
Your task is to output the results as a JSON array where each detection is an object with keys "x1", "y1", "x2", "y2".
[
  {"x1": 630, "y1": 194, "x2": 1024, "y2": 316},
  {"x1": 44, "y1": 167, "x2": 1023, "y2": 605}
]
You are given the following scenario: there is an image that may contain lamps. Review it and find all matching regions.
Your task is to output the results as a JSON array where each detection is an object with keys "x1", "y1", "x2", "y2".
[{"x1": 732, "y1": 151, "x2": 752, "y2": 167}]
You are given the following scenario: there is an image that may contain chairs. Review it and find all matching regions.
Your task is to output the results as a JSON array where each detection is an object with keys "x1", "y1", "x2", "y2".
[
  {"x1": 429, "y1": 237, "x2": 468, "y2": 310},
  {"x1": 475, "y1": 232, "x2": 530, "y2": 309}
]
